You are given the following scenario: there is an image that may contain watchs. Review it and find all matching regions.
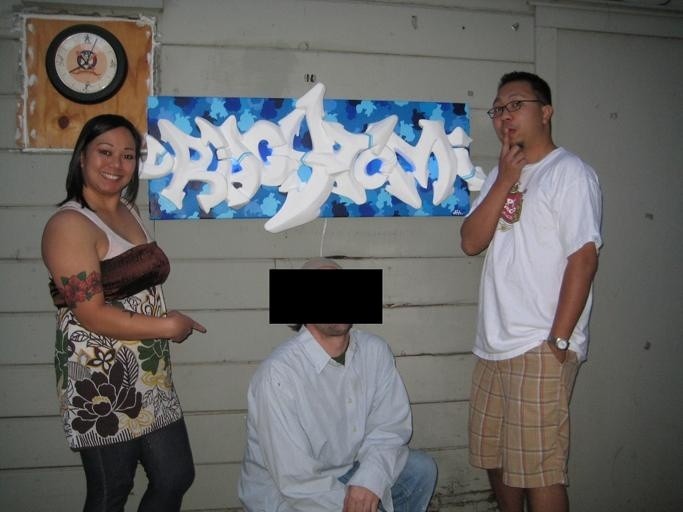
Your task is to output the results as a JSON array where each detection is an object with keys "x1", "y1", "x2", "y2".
[{"x1": 547, "y1": 334, "x2": 569, "y2": 350}]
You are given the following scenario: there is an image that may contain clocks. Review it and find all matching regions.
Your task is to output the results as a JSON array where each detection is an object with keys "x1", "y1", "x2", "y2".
[{"x1": 44, "y1": 21, "x2": 128, "y2": 106}]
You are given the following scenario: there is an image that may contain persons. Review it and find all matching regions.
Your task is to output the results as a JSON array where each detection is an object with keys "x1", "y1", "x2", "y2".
[
  {"x1": 38, "y1": 112, "x2": 209, "y2": 510},
  {"x1": 459, "y1": 70, "x2": 604, "y2": 511},
  {"x1": 235, "y1": 257, "x2": 441, "y2": 511}
]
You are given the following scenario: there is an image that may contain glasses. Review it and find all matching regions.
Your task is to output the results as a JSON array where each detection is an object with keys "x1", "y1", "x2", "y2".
[{"x1": 488, "y1": 97, "x2": 547, "y2": 120}]
